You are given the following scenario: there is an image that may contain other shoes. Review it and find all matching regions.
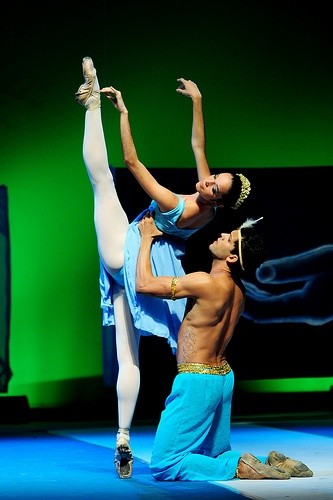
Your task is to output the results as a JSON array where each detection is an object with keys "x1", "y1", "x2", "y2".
[
  {"x1": 241, "y1": 450, "x2": 290, "y2": 480},
  {"x1": 74, "y1": 57, "x2": 96, "y2": 103},
  {"x1": 113, "y1": 438, "x2": 133, "y2": 480},
  {"x1": 269, "y1": 453, "x2": 312, "y2": 480}
]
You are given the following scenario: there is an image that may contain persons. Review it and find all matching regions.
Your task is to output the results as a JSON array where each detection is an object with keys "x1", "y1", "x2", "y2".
[
  {"x1": 131, "y1": 215, "x2": 312, "y2": 481},
  {"x1": 76, "y1": 56, "x2": 252, "y2": 478}
]
